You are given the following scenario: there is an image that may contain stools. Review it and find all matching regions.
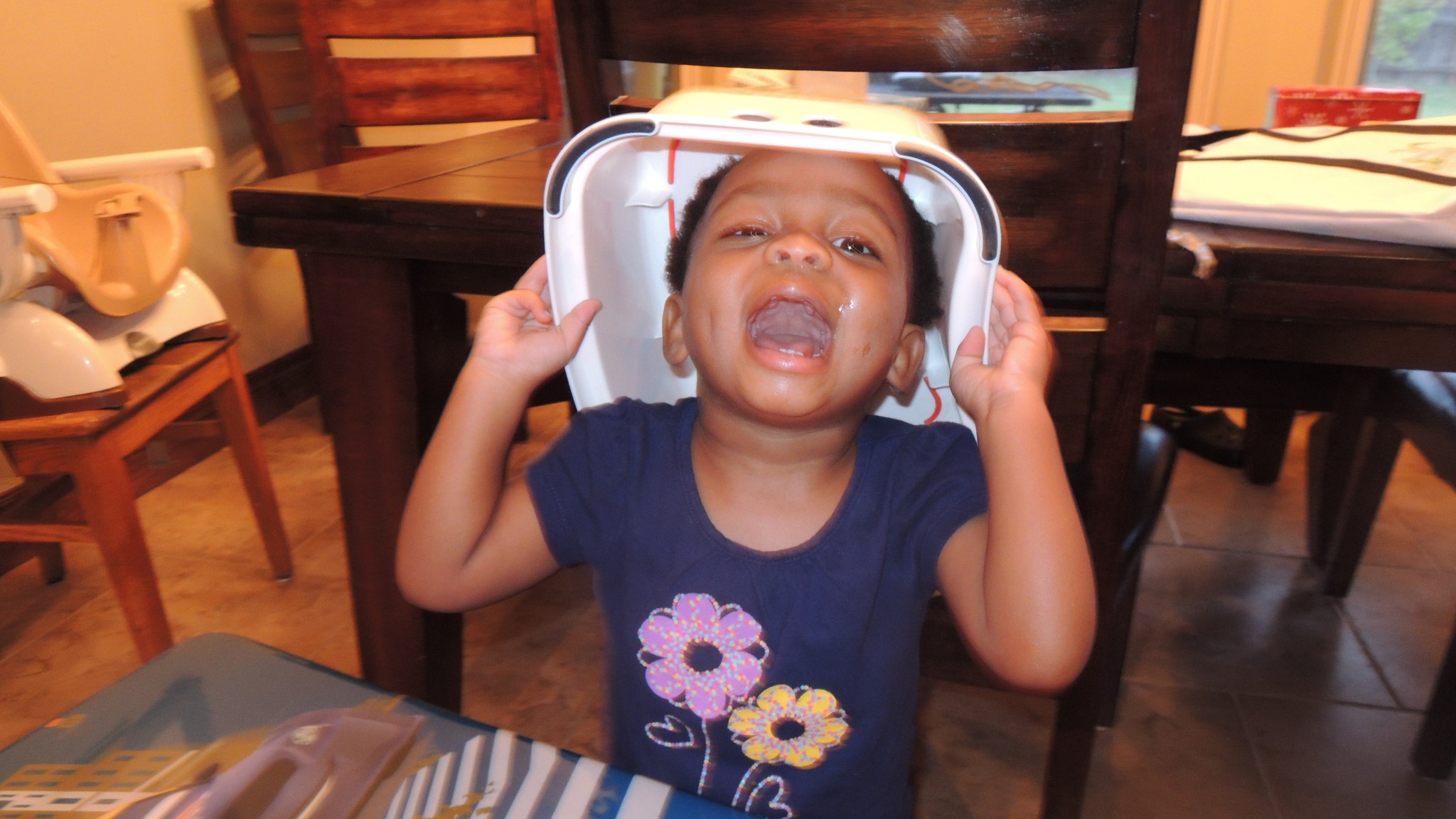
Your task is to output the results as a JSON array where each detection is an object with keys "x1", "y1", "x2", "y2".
[{"x1": 1322, "y1": 369, "x2": 1456, "y2": 779}]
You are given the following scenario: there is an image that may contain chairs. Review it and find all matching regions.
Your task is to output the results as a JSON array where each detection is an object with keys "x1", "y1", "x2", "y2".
[
  {"x1": 0, "y1": 320, "x2": 292, "y2": 664},
  {"x1": 210, "y1": 0, "x2": 563, "y2": 176},
  {"x1": 553, "y1": 0, "x2": 1203, "y2": 819}
]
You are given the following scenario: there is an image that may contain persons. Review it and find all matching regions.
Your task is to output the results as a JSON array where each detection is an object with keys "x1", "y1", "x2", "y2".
[{"x1": 397, "y1": 148, "x2": 1096, "y2": 819}]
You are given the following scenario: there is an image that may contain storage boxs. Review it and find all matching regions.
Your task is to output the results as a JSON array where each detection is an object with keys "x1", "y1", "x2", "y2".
[
  {"x1": 1267, "y1": 86, "x2": 1422, "y2": 126},
  {"x1": 542, "y1": 90, "x2": 1001, "y2": 439}
]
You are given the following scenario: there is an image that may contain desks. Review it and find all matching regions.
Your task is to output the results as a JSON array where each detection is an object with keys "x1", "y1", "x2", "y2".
[
  {"x1": 1154, "y1": 221, "x2": 1456, "y2": 782},
  {"x1": 232, "y1": 126, "x2": 1456, "y2": 713},
  {"x1": 0, "y1": 631, "x2": 747, "y2": 819}
]
